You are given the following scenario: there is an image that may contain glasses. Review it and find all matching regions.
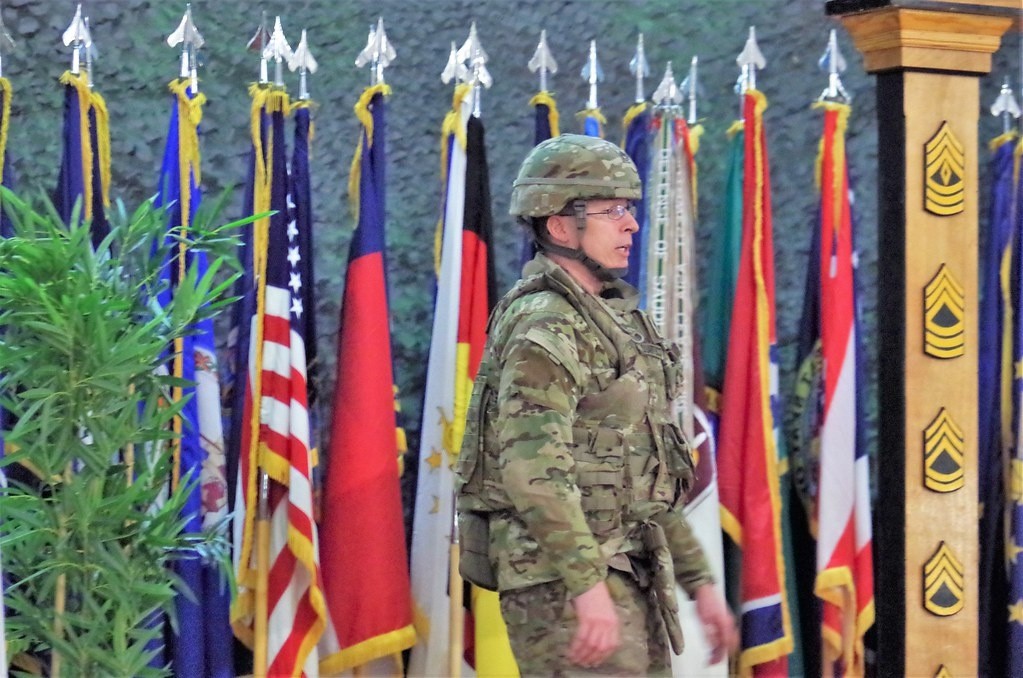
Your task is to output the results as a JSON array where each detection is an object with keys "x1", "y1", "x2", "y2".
[{"x1": 558, "y1": 203, "x2": 638, "y2": 220}]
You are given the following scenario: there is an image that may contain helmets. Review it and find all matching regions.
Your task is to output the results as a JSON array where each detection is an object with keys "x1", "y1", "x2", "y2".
[{"x1": 509, "y1": 133, "x2": 642, "y2": 217}]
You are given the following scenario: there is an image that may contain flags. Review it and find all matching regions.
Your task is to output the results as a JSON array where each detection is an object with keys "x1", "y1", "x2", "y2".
[{"x1": 0, "y1": 73, "x2": 1023, "y2": 678}]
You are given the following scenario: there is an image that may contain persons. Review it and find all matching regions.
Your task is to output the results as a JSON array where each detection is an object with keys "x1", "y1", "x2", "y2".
[{"x1": 450, "y1": 134, "x2": 741, "y2": 678}]
